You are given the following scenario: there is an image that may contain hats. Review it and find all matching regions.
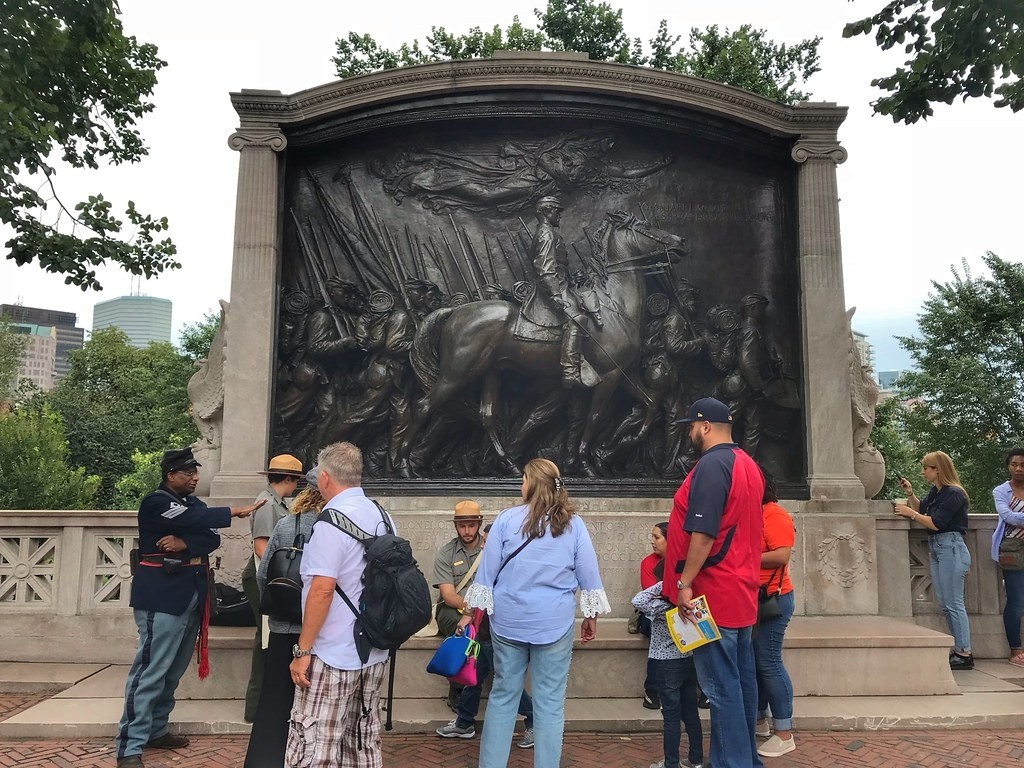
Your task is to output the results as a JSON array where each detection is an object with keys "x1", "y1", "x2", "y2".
[
  {"x1": 446, "y1": 501, "x2": 490, "y2": 522},
  {"x1": 257, "y1": 455, "x2": 306, "y2": 477},
  {"x1": 160, "y1": 447, "x2": 202, "y2": 478},
  {"x1": 675, "y1": 397, "x2": 734, "y2": 423}
]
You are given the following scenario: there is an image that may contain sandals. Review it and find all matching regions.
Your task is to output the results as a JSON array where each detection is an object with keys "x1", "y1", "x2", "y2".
[{"x1": 1008, "y1": 651, "x2": 1024, "y2": 667}]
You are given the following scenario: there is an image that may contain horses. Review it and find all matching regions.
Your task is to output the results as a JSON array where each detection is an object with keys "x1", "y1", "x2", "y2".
[{"x1": 388, "y1": 208, "x2": 690, "y2": 481}]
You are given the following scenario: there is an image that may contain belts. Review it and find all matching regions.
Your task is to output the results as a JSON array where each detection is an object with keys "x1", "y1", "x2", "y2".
[{"x1": 143, "y1": 554, "x2": 207, "y2": 565}]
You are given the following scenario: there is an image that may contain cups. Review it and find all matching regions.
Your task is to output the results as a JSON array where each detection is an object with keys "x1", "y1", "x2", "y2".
[{"x1": 895, "y1": 499, "x2": 908, "y2": 507}]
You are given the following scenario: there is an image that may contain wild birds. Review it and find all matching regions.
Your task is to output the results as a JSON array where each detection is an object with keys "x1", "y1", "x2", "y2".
[
  {"x1": 844, "y1": 307, "x2": 880, "y2": 456},
  {"x1": 187, "y1": 299, "x2": 230, "y2": 452}
]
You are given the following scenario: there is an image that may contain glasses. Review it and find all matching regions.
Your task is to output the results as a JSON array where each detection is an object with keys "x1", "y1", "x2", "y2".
[{"x1": 177, "y1": 471, "x2": 199, "y2": 476}]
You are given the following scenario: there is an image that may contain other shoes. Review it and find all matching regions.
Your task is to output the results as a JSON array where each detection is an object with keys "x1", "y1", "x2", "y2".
[
  {"x1": 697, "y1": 688, "x2": 711, "y2": 708},
  {"x1": 643, "y1": 686, "x2": 660, "y2": 709}
]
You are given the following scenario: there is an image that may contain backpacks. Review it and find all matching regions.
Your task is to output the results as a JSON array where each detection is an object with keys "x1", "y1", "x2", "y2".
[
  {"x1": 307, "y1": 499, "x2": 431, "y2": 662},
  {"x1": 209, "y1": 582, "x2": 257, "y2": 627},
  {"x1": 259, "y1": 513, "x2": 304, "y2": 624}
]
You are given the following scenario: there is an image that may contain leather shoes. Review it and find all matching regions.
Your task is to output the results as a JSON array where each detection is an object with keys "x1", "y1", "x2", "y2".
[
  {"x1": 116, "y1": 754, "x2": 145, "y2": 768},
  {"x1": 144, "y1": 732, "x2": 190, "y2": 749},
  {"x1": 949, "y1": 650, "x2": 974, "y2": 670}
]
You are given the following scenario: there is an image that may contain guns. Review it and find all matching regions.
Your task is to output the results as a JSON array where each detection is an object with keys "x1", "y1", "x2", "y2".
[{"x1": 288, "y1": 163, "x2": 699, "y2": 350}]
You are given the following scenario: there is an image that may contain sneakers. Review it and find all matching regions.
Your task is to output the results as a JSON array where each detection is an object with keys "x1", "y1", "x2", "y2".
[
  {"x1": 435, "y1": 719, "x2": 475, "y2": 738},
  {"x1": 756, "y1": 722, "x2": 771, "y2": 736},
  {"x1": 516, "y1": 727, "x2": 535, "y2": 747},
  {"x1": 650, "y1": 759, "x2": 665, "y2": 768},
  {"x1": 680, "y1": 758, "x2": 704, "y2": 768},
  {"x1": 757, "y1": 733, "x2": 795, "y2": 757}
]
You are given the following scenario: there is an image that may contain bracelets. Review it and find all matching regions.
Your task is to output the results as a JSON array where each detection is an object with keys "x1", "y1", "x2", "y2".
[
  {"x1": 907, "y1": 493, "x2": 913, "y2": 496},
  {"x1": 911, "y1": 512, "x2": 919, "y2": 520},
  {"x1": 463, "y1": 610, "x2": 474, "y2": 617},
  {"x1": 677, "y1": 580, "x2": 690, "y2": 589}
]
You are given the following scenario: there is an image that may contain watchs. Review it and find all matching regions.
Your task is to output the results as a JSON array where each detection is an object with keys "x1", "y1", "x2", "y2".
[{"x1": 292, "y1": 643, "x2": 313, "y2": 657}]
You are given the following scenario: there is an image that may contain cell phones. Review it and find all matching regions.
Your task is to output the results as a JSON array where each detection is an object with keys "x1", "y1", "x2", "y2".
[{"x1": 896, "y1": 473, "x2": 907, "y2": 487}]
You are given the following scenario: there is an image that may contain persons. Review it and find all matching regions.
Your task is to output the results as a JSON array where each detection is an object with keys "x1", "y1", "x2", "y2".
[
  {"x1": 115, "y1": 448, "x2": 268, "y2": 768},
  {"x1": 454, "y1": 458, "x2": 611, "y2": 768},
  {"x1": 496, "y1": 283, "x2": 787, "y2": 479},
  {"x1": 282, "y1": 441, "x2": 395, "y2": 768},
  {"x1": 894, "y1": 451, "x2": 974, "y2": 669},
  {"x1": 632, "y1": 522, "x2": 704, "y2": 768},
  {"x1": 756, "y1": 462, "x2": 795, "y2": 757},
  {"x1": 431, "y1": 500, "x2": 535, "y2": 746},
  {"x1": 529, "y1": 196, "x2": 603, "y2": 389},
  {"x1": 660, "y1": 396, "x2": 765, "y2": 768},
  {"x1": 273, "y1": 278, "x2": 531, "y2": 478},
  {"x1": 991, "y1": 448, "x2": 1024, "y2": 667},
  {"x1": 244, "y1": 455, "x2": 328, "y2": 768}
]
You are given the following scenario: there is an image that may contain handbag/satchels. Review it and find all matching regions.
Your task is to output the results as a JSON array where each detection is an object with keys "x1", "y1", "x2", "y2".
[
  {"x1": 426, "y1": 624, "x2": 475, "y2": 677},
  {"x1": 446, "y1": 623, "x2": 481, "y2": 687},
  {"x1": 758, "y1": 586, "x2": 784, "y2": 623},
  {"x1": 628, "y1": 609, "x2": 640, "y2": 634},
  {"x1": 999, "y1": 538, "x2": 1024, "y2": 571}
]
